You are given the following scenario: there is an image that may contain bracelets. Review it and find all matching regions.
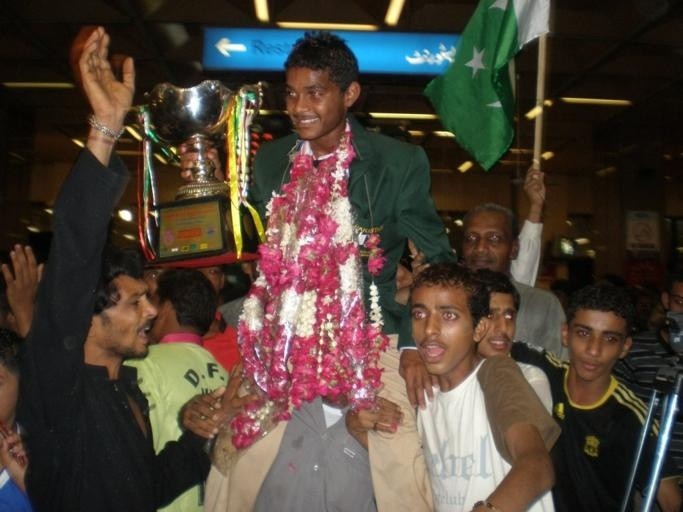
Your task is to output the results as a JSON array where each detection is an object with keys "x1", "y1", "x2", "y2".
[{"x1": 89, "y1": 116, "x2": 126, "y2": 144}]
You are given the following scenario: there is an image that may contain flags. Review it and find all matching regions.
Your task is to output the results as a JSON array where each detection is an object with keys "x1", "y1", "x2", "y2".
[{"x1": 423, "y1": 3, "x2": 555, "y2": 172}]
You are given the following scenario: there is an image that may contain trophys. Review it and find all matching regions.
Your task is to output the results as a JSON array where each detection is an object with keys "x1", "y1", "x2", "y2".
[{"x1": 122, "y1": 77, "x2": 269, "y2": 278}]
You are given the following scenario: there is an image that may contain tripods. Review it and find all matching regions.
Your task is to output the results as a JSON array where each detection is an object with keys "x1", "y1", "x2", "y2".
[{"x1": 620, "y1": 356, "x2": 683, "y2": 512}]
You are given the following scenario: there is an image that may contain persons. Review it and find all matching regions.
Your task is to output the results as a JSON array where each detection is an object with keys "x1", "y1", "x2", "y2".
[
  {"x1": 2, "y1": 244, "x2": 376, "y2": 512},
  {"x1": 17, "y1": 25, "x2": 229, "y2": 511},
  {"x1": 346, "y1": 167, "x2": 682, "y2": 511},
  {"x1": 173, "y1": 30, "x2": 461, "y2": 408}
]
